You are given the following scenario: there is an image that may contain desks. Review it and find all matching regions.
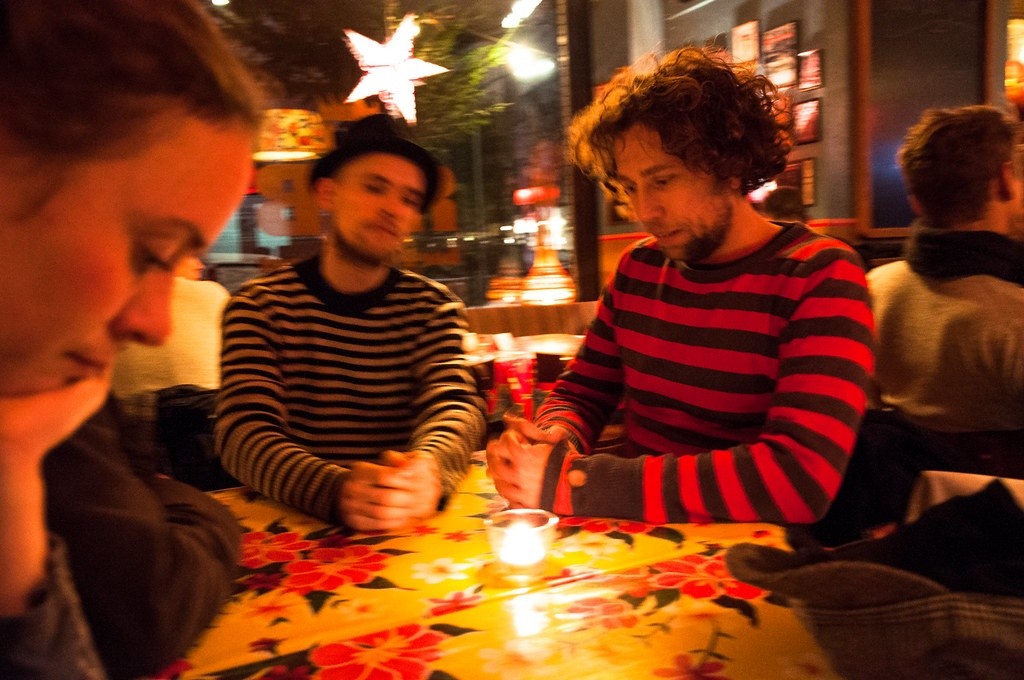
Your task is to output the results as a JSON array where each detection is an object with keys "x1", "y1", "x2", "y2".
[{"x1": 162, "y1": 448, "x2": 840, "y2": 680}]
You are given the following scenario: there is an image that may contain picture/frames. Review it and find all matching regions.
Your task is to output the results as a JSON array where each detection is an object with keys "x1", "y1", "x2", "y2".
[
  {"x1": 784, "y1": 155, "x2": 819, "y2": 207},
  {"x1": 789, "y1": 97, "x2": 823, "y2": 145},
  {"x1": 795, "y1": 47, "x2": 824, "y2": 93},
  {"x1": 759, "y1": 19, "x2": 800, "y2": 94},
  {"x1": 728, "y1": 18, "x2": 761, "y2": 64}
]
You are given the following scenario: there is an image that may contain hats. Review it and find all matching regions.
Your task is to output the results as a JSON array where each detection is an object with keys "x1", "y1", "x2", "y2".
[
  {"x1": 310, "y1": 113, "x2": 437, "y2": 215},
  {"x1": 726, "y1": 542, "x2": 1024, "y2": 680}
]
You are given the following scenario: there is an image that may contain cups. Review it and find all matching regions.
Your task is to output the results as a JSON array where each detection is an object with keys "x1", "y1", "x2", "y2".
[{"x1": 481, "y1": 507, "x2": 560, "y2": 583}]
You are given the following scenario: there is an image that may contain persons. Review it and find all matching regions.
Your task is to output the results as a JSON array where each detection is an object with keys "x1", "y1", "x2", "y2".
[
  {"x1": 0, "y1": 0, "x2": 1024, "y2": 680},
  {"x1": 214, "y1": 112, "x2": 489, "y2": 531}
]
